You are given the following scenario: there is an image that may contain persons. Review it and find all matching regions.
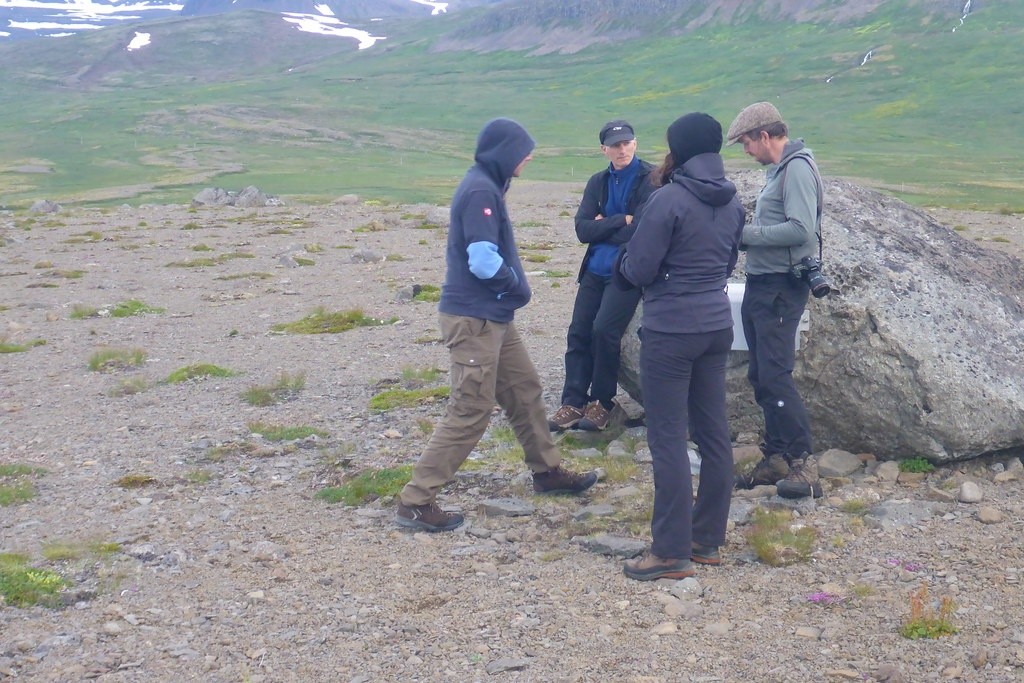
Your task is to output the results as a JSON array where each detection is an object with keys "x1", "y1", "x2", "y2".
[
  {"x1": 727, "y1": 100, "x2": 827, "y2": 498},
  {"x1": 546, "y1": 118, "x2": 662, "y2": 431},
  {"x1": 616, "y1": 111, "x2": 744, "y2": 582},
  {"x1": 398, "y1": 119, "x2": 596, "y2": 532}
]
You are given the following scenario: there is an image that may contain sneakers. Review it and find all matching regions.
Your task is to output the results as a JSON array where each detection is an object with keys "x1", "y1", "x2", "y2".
[
  {"x1": 532, "y1": 463, "x2": 598, "y2": 493},
  {"x1": 690, "y1": 539, "x2": 721, "y2": 565},
  {"x1": 735, "y1": 439, "x2": 790, "y2": 488},
  {"x1": 396, "y1": 501, "x2": 464, "y2": 531},
  {"x1": 776, "y1": 452, "x2": 822, "y2": 498},
  {"x1": 547, "y1": 405, "x2": 586, "y2": 431},
  {"x1": 624, "y1": 547, "x2": 694, "y2": 580},
  {"x1": 578, "y1": 399, "x2": 617, "y2": 431}
]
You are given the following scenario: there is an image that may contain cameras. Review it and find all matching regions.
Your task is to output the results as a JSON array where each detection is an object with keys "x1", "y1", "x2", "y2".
[{"x1": 788, "y1": 256, "x2": 830, "y2": 298}]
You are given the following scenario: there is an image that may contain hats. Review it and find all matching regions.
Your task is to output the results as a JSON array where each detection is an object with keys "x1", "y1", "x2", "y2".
[
  {"x1": 668, "y1": 112, "x2": 722, "y2": 166},
  {"x1": 599, "y1": 119, "x2": 635, "y2": 147},
  {"x1": 726, "y1": 102, "x2": 781, "y2": 147}
]
